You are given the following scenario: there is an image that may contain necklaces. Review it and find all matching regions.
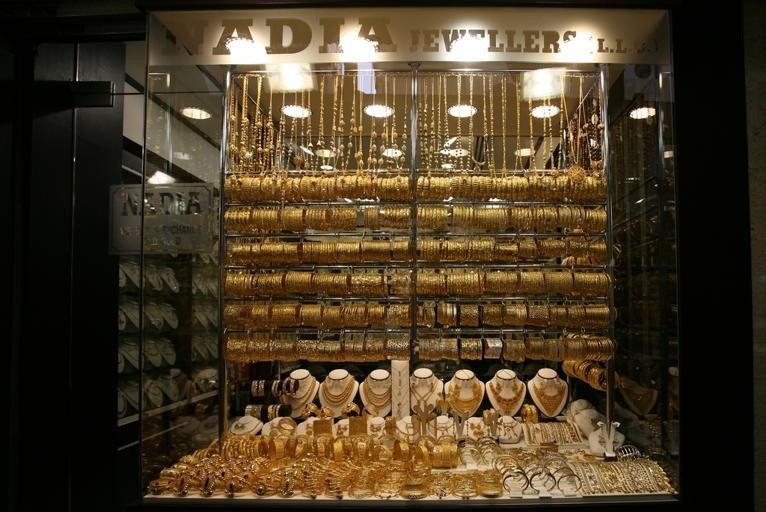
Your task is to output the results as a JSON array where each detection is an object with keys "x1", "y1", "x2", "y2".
[
  {"x1": 619, "y1": 370, "x2": 676, "y2": 459},
  {"x1": 570, "y1": 457, "x2": 677, "y2": 496},
  {"x1": 118, "y1": 244, "x2": 220, "y2": 422},
  {"x1": 227, "y1": 416, "x2": 263, "y2": 436}
]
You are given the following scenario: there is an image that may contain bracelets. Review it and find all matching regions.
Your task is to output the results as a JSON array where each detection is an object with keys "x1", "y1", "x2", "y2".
[
  {"x1": 563, "y1": 364, "x2": 610, "y2": 392},
  {"x1": 192, "y1": 435, "x2": 458, "y2": 469},
  {"x1": 243, "y1": 378, "x2": 299, "y2": 422},
  {"x1": 450, "y1": 437, "x2": 581, "y2": 501}
]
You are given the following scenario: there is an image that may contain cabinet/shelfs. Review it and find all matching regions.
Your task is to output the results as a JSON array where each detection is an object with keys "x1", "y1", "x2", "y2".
[
  {"x1": 221, "y1": 76, "x2": 610, "y2": 362},
  {"x1": 609, "y1": 79, "x2": 676, "y2": 384},
  {"x1": 117, "y1": 235, "x2": 219, "y2": 424}
]
